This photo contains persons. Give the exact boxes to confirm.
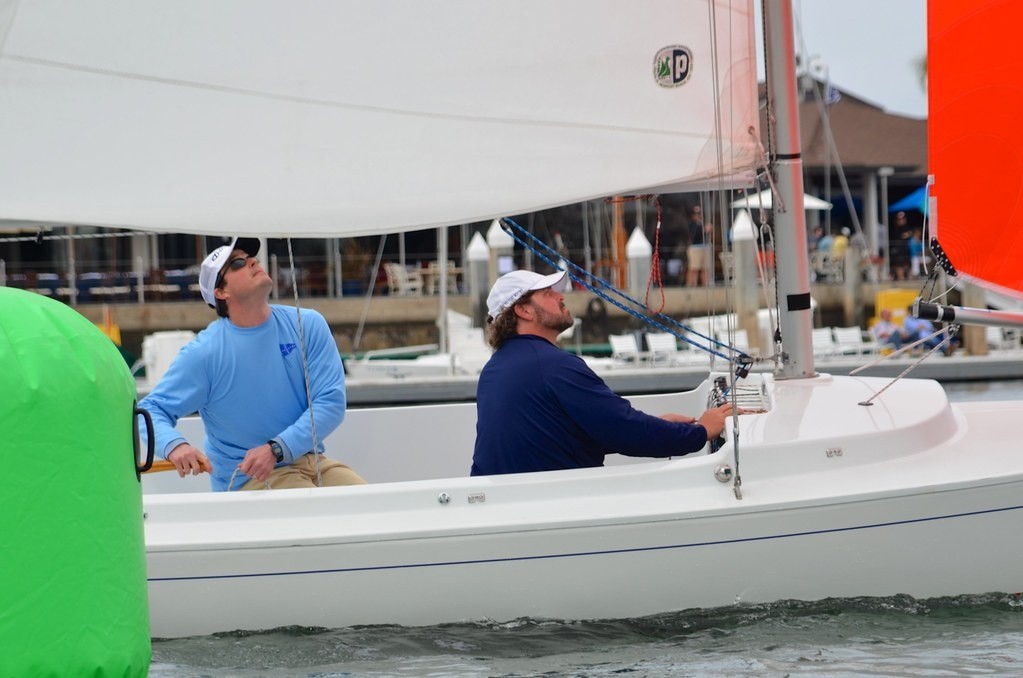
[134,236,366,492]
[469,269,741,477]
[685,202,927,282]
[872,302,959,357]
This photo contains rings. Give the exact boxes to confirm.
[249,470,255,475]
[253,474,258,480]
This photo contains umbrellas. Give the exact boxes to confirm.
[889,185,928,214]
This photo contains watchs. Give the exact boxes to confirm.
[266,440,284,463]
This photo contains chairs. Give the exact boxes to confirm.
[384,258,460,297]
[607,324,880,371]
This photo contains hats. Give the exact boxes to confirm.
[486,270,568,325]
[199,237,260,309]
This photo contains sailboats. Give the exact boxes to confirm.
[0,0,1023,641]
[335,0,1023,411]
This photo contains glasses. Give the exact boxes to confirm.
[215,249,257,289]
